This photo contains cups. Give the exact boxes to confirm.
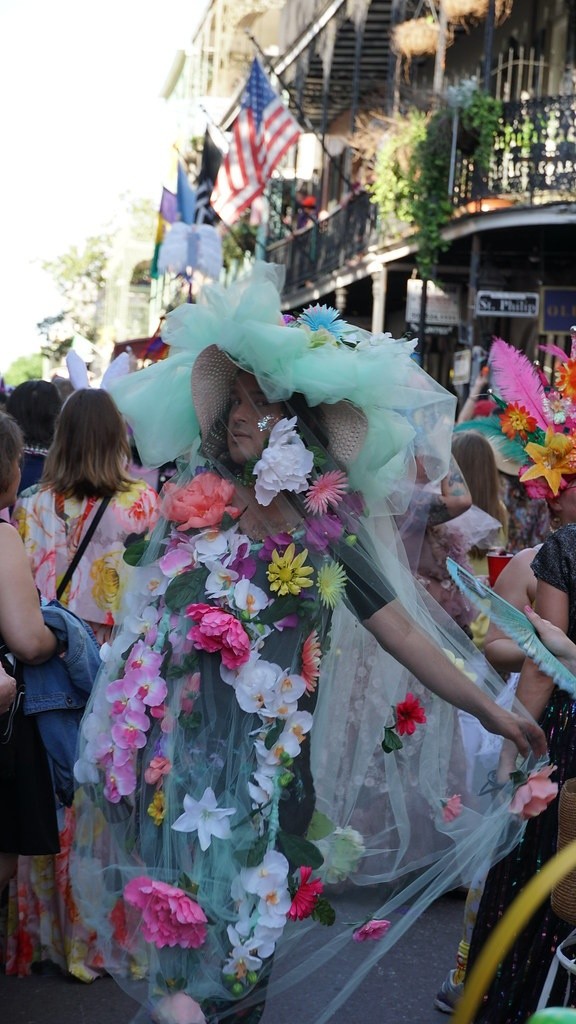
[487,552,514,587]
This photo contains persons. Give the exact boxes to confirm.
[0,255,575,1024]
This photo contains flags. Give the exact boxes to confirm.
[212,61,304,227]
[192,131,224,226]
[174,166,203,268]
[150,189,186,281]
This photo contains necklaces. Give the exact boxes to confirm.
[233,474,254,488]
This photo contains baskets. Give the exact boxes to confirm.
[550,777,576,925]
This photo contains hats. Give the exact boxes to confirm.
[190,306,369,481]
[488,435,528,475]
[454,327,576,500]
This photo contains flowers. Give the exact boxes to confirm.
[471,327,576,500]
[67,415,558,996]
[282,302,394,351]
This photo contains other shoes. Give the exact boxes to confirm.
[433,969,462,1014]
